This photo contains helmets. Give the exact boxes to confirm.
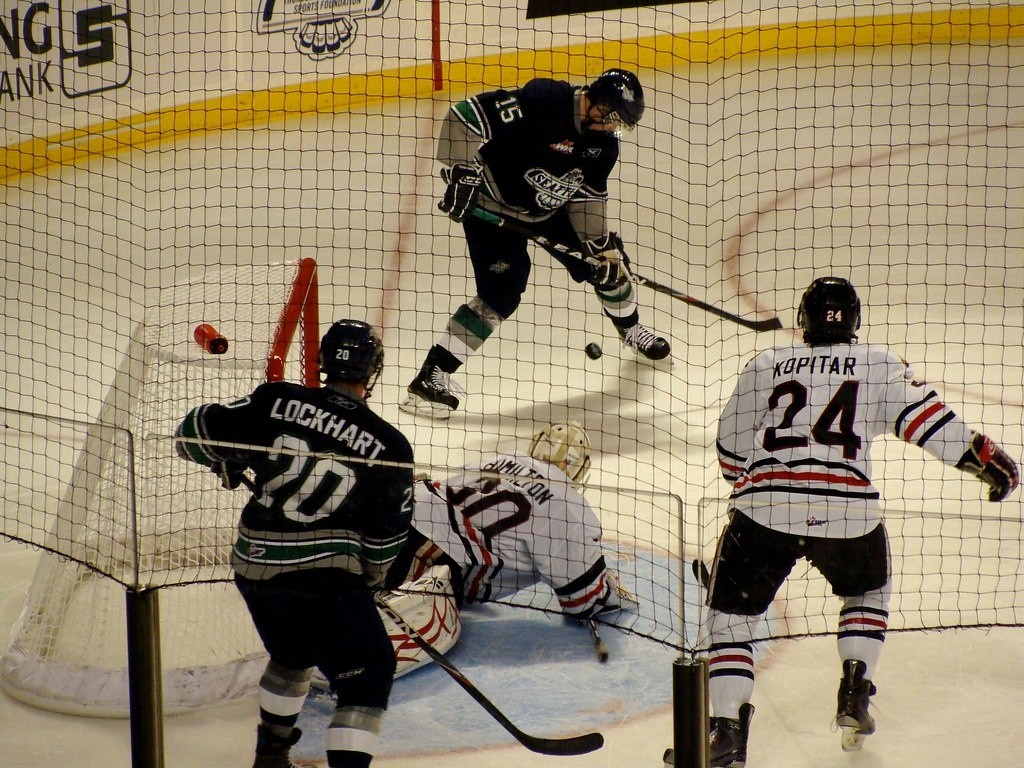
[798,277,861,335]
[316,319,384,391]
[586,68,645,136]
[528,420,591,484]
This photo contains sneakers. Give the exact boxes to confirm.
[252,723,317,768]
[398,345,459,420]
[709,703,755,768]
[837,659,876,751]
[614,323,675,367]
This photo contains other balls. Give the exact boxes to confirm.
[586,343,602,360]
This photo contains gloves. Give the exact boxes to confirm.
[582,231,630,287]
[214,461,248,490]
[437,162,483,223]
[962,433,1019,502]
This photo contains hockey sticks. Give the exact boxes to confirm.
[690,559,710,589]
[208,459,606,757]
[587,619,609,663]
[470,204,801,332]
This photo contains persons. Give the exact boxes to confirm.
[174,318,416,768]
[309,422,638,695]
[663,276,1019,768]
[398,69,672,418]
